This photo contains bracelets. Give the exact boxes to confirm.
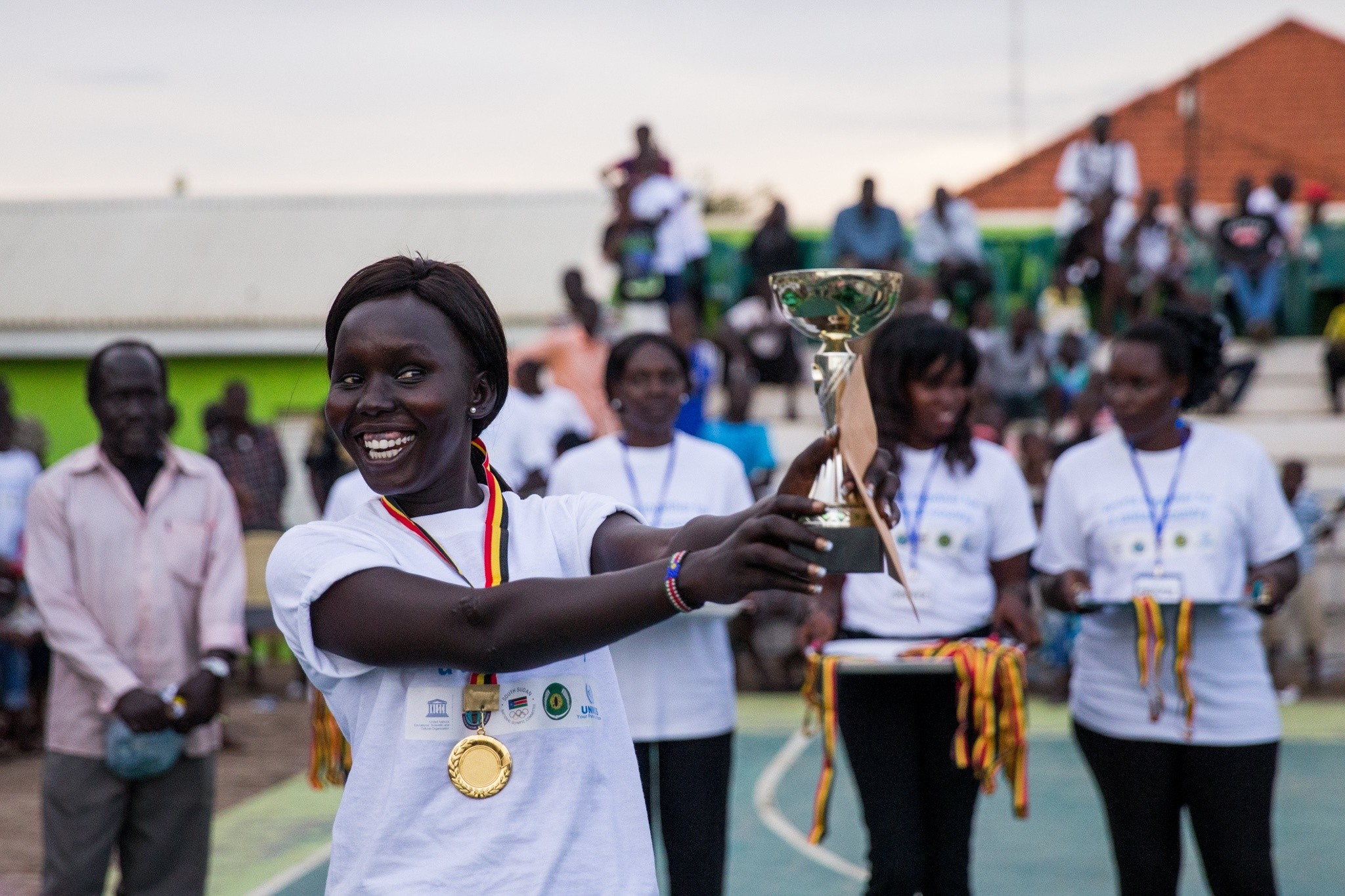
[664,549,705,613]
[196,656,229,682]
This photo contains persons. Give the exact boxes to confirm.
[6,114,1345,896]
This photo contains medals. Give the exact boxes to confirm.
[448,728,512,799]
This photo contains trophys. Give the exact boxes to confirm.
[770,268,904,573]
